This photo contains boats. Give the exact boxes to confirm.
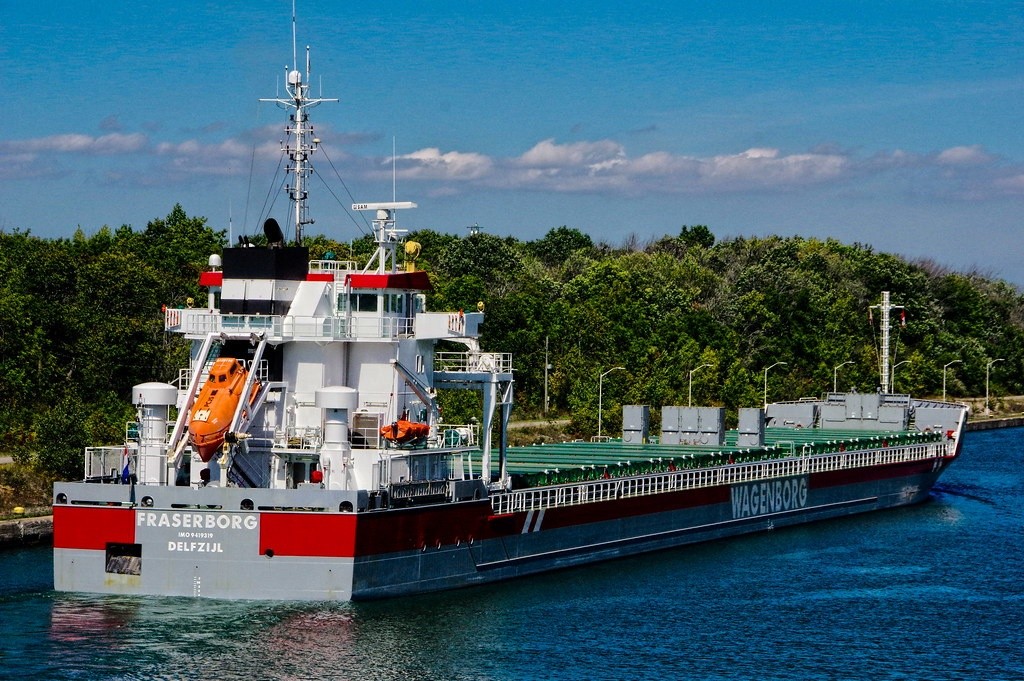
[52,1,969,602]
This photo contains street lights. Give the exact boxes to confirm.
[689,365,712,405]
[834,362,853,394]
[891,361,912,394]
[764,362,786,412]
[943,360,962,403]
[599,367,624,443]
[986,359,1003,414]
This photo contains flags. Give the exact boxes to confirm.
[122,447,129,481]
[901,311,906,328]
